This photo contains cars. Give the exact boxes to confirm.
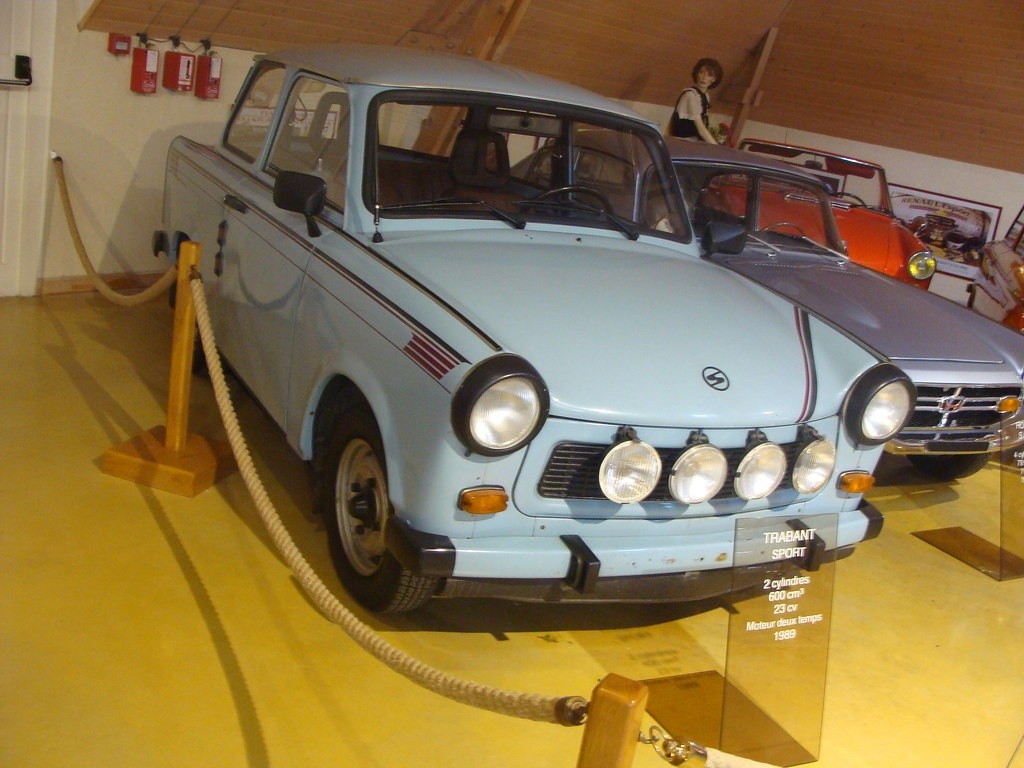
[698,136,939,292]
[152,40,915,619]
[913,214,956,244]
[509,137,1024,483]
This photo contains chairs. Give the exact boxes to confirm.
[446,127,532,213]
[305,89,408,206]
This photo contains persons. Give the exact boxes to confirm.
[671,58,723,145]
